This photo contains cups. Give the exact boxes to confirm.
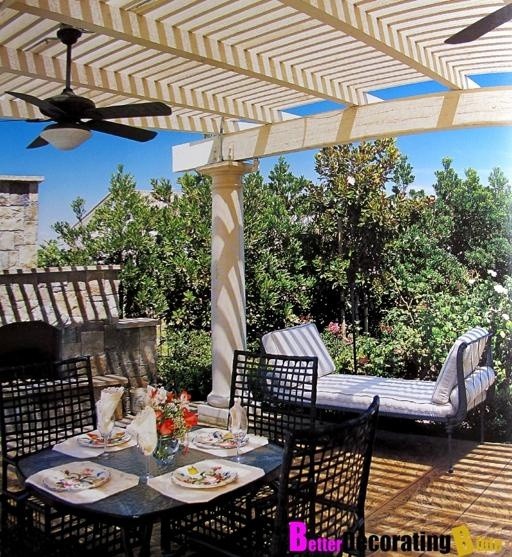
[229,415,247,461]
[137,431,157,483]
[98,415,115,460]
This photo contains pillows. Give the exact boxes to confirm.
[431,327,490,404]
[261,324,337,380]
[451,367,494,407]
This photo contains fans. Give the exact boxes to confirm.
[5,25,172,149]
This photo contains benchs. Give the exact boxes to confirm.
[254,323,497,473]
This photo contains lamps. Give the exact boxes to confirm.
[39,28,95,150]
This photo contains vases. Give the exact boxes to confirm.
[150,435,179,476]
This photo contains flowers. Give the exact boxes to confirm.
[144,388,197,461]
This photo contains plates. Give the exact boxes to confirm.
[192,430,243,450]
[171,462,238,489]
[76,432,131,448]
[42,465,111,492]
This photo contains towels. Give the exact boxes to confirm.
[229,400,249,435]
[96,387,124,430]
[127,407,157,455]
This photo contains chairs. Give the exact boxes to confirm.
[0,355,142,555]
[164,396,381,556]
[168,350,319,521]
